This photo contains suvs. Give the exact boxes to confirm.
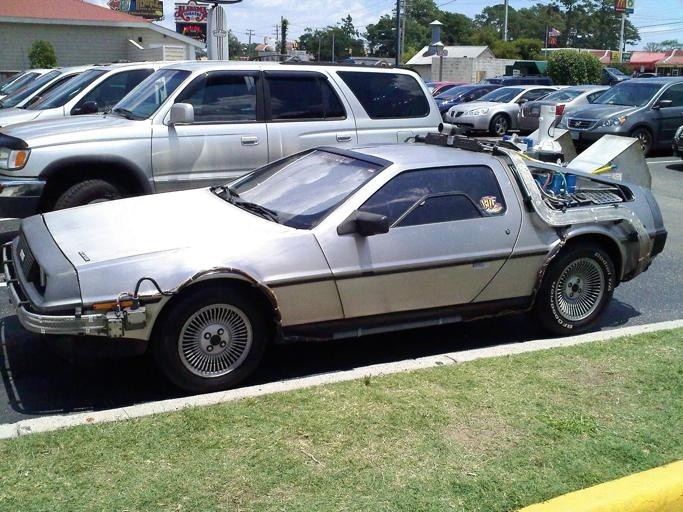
[0,62,112,111]
[560,74,683,156]
[0,60,447,221]
[0,61,283,128]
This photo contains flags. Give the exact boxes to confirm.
[548,27,561,37]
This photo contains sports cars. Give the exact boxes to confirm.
[0,135,665,397]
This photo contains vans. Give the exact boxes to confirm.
[481,75,552,86]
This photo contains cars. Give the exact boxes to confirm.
[635,72,656,78]
[602,67,629,84]
[432,84,505,116]
[424,81,467,97]
[515,84,610,134]
[671,126,682,161]
[442,83,558,136]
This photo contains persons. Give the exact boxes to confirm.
[630,68,639,78]
